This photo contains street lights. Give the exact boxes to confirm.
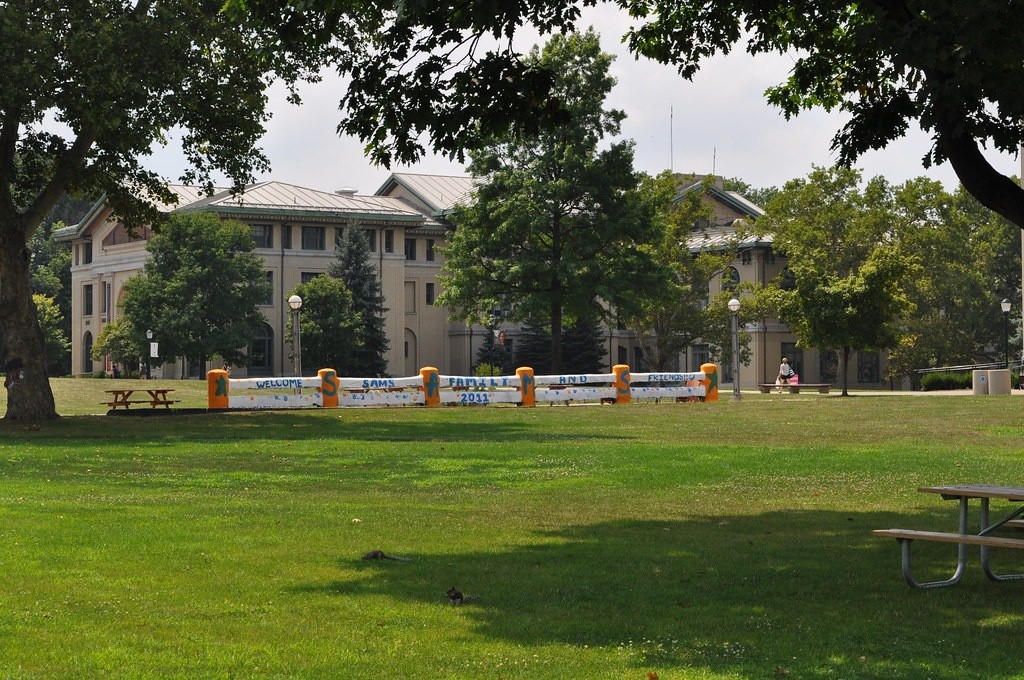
[1001,298,1011,368]
[287,295,304,393]
[728,299,742,400]
[146,329,153,378]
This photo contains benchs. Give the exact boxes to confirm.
[871,528,1024,554]
[101,399,177,407]
[755,383,832,395]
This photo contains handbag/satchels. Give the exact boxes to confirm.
[774,378,784,390]
[789,368,794,377]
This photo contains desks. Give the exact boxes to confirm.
[872,483,1024,594]
[99,388,178,414]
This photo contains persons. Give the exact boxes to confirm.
[112,365,118,379]
[222,362,228,371]
[777,357,791,393]
[227,363,231,377]
[139,362,147,379]
